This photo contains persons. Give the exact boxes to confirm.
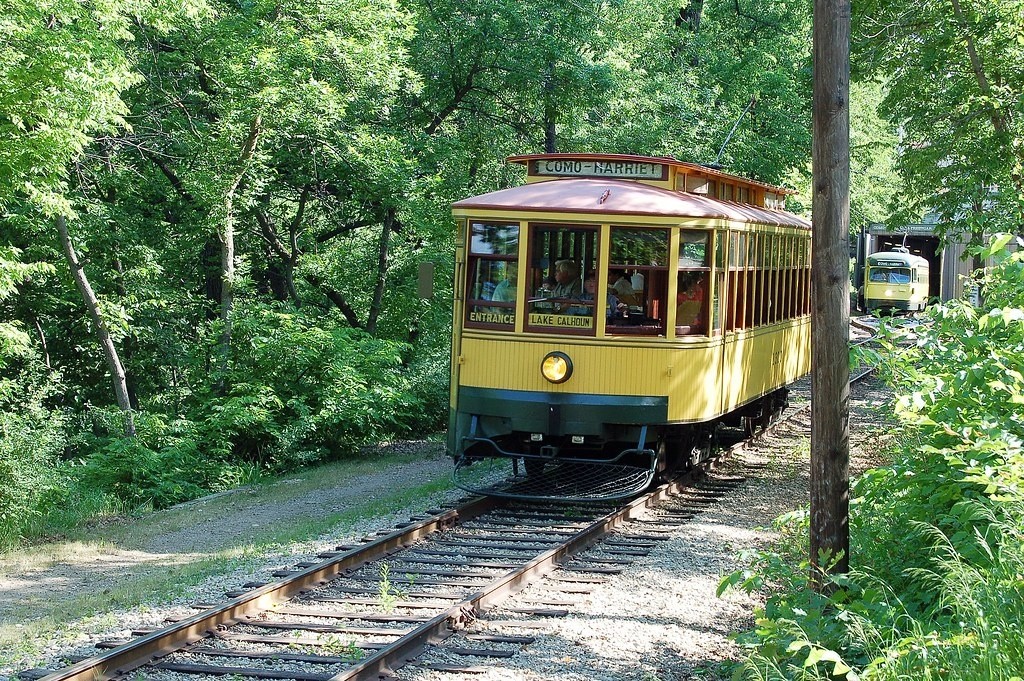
[490,263,518,314]
[542,260,644,316]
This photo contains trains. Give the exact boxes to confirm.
[445,97,813,502]
[863,233,930,314]
